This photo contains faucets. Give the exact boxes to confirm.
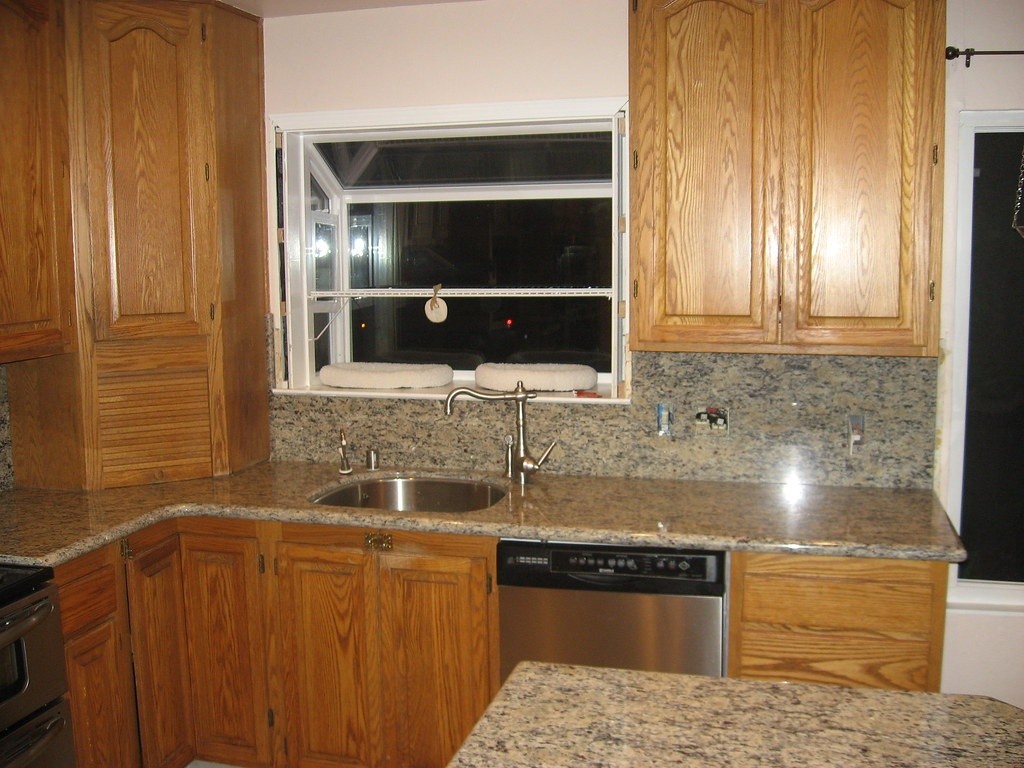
[444,381,556,484]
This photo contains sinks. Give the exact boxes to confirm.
[311,475,508,512]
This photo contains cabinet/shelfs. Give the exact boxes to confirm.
[274,520,493,767]
[57,520,194,768]
[628,1,948,358]
[729,552,947,690]
[184,519,273,767]
[1,1,272,494]
